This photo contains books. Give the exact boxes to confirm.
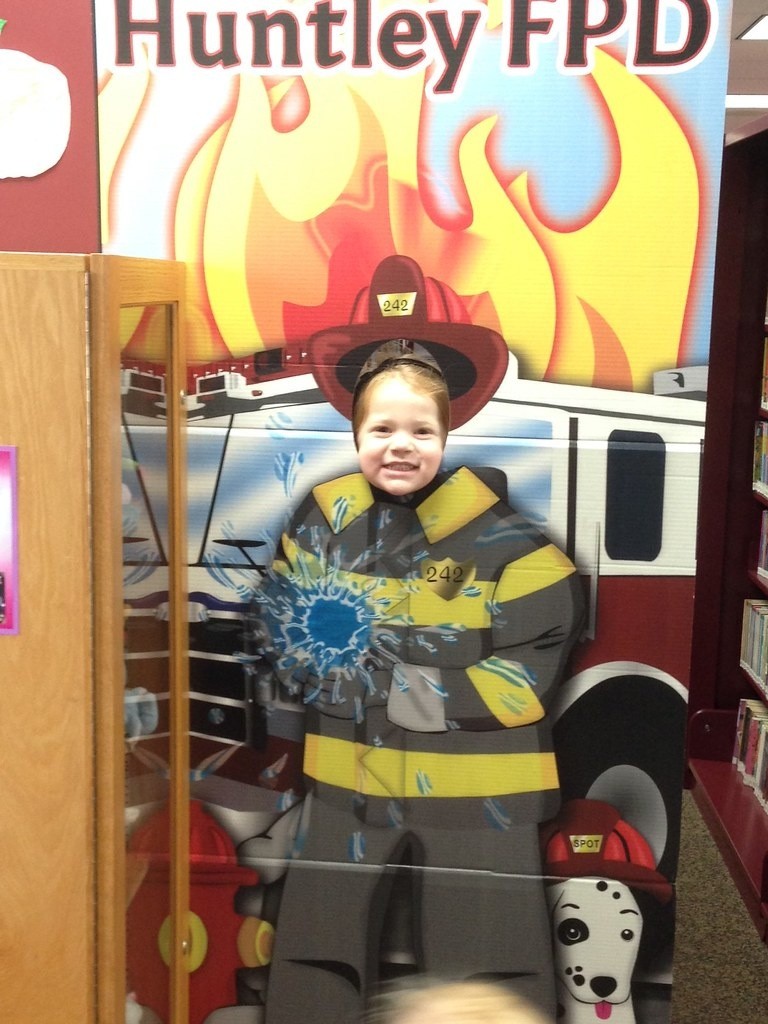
[730,699,768,812]
[756,511,767,578]
[739,600,768,692]
[759,296,768,415]
[753,422,768,497]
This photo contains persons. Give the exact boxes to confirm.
[352,360,451,496]
